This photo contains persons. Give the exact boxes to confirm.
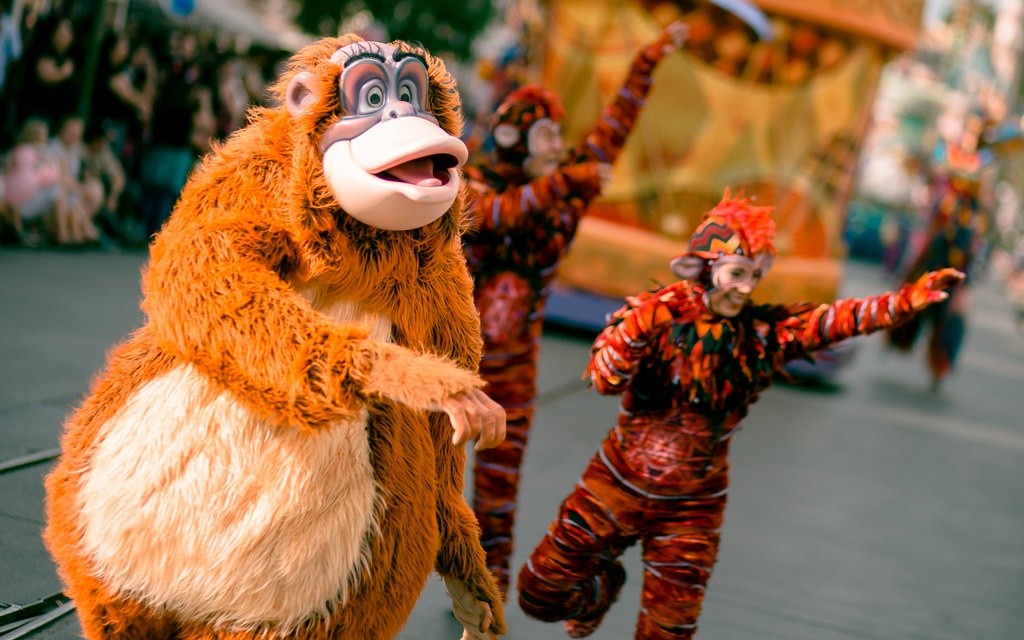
[866,90,1006,398]
[459,22,693,607]
[516,185,967,640]
[0,0,288,251]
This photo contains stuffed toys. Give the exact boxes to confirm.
[40,32,517,640]
